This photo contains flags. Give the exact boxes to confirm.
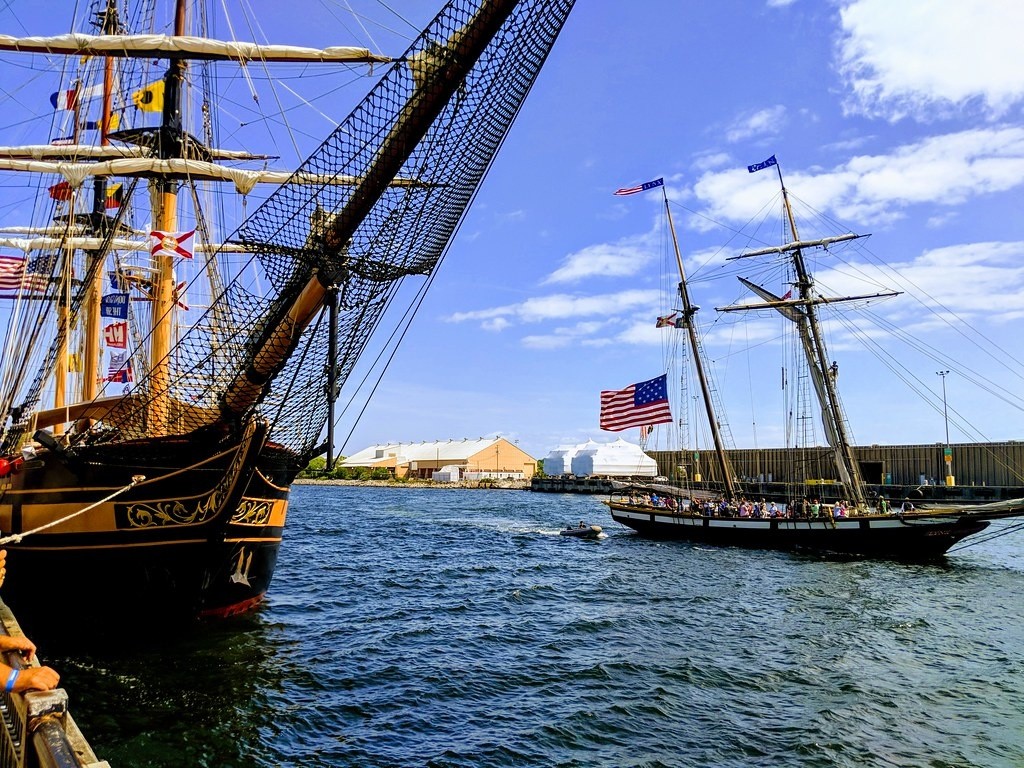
[599,374,673,432]
[747,154,779,174]
[655,311,677,329]
[614,178,664,196]
[781,290,791,301]
[0,77,196,384]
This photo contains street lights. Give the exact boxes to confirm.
[691,395,699,474]
[935,370,952,476]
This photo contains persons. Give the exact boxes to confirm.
[579,521,586,529]
[828,361,839,389]
[629,491,914,519]
[0,548,60,692]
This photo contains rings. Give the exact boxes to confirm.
[0,570,5,577]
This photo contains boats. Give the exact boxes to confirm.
[0,0,579,629]
[599,154,992,561]
[559,525,602,539]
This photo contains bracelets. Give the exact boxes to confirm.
[5,670,19,692]
